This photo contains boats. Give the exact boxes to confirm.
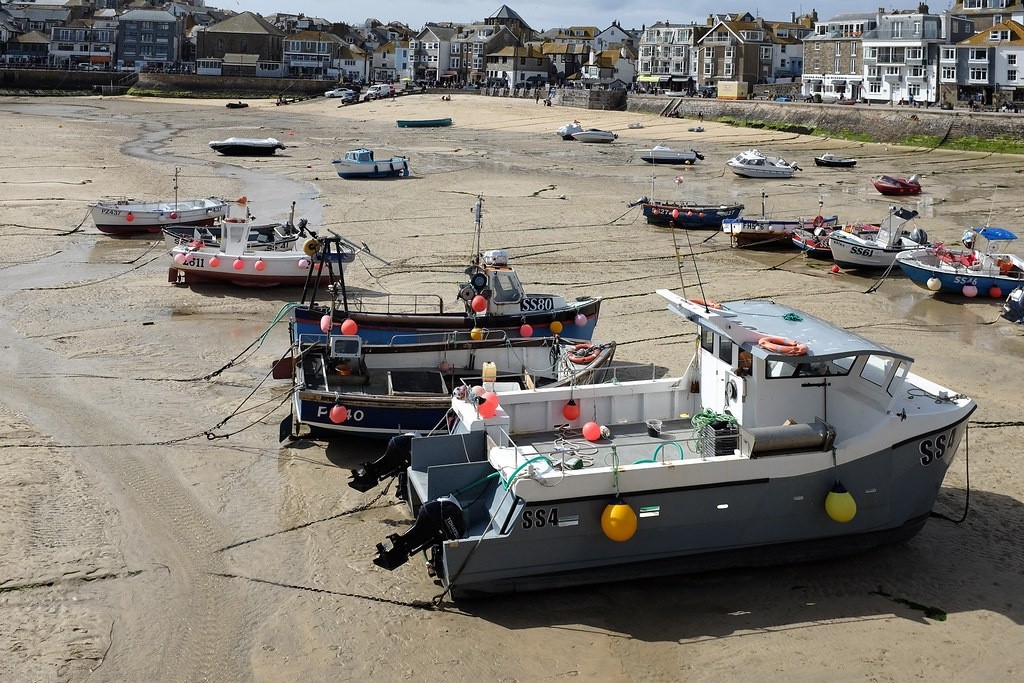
[776,97,792,102]
[688,126,704,132]
[827,203,940,275]
[628,122,645,129]
[870,172,923,196]
[160,198,308,259]
[396,116,453,128]
[605,149,746,231]
[838,99,856,105]
[394,215,979,606]
[571,129,618,145]
[720,186,838,252]
[208,137,287,157]
[894,181,1024,327]
[632,140,707,165]
[813,152,859,169]
[287,190,603,350]
[334,143,417,181]
[665,87,689,98]
[226,101,249,108]
[271,328,618,450]
[790,192,911,261]
[168,217,369,292]
[729,151,804,179]
[86,164,229,236]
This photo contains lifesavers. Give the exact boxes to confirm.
[812,215,824,228]
[567,343,600,363]
[759,336,809,355]
[688,298,723,310]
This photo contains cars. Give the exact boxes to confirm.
[324,87,353,98]
[78,62,118,72]
[941,102,954,110]
[340,90,360,104]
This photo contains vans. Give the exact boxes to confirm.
[367,84,391,101]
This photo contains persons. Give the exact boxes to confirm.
[442,95,451,101]
[535,94,551,106]
[1003,100,1014,109]
[383,78,393,85]
[698,113,703,122]
[354,76,365,89]
[703,90,711,98]
[631,84,635,94]
[908,94,913,107]
[968,99,979,111]
[443,81,473,89]
[405,81,408,90]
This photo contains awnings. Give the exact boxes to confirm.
[803,79,861,85]
